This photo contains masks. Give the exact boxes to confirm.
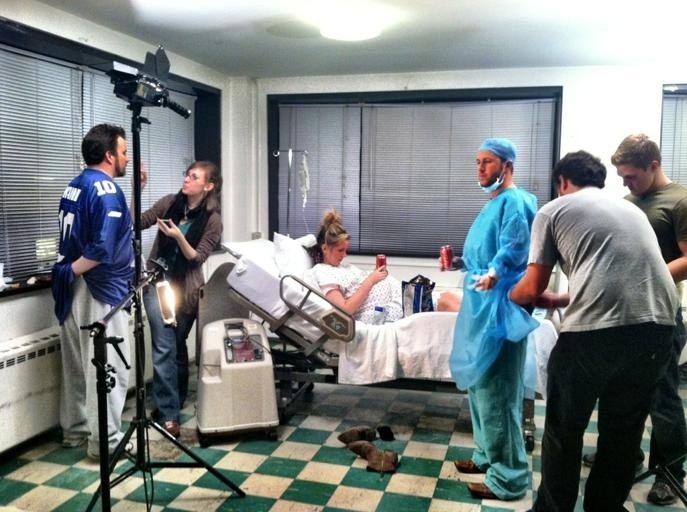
[478,169,504,193]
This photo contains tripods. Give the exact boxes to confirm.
[84,298,246,512]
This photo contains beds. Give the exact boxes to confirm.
[200,237,561,453]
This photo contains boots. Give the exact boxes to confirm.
[348,442,399,473]
[338,426,394,444]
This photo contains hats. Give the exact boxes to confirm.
[481,138,517,163]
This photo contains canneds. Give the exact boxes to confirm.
[440,245,453,269]
[376,253,386,270]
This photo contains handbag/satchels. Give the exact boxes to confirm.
[401,275,435,317]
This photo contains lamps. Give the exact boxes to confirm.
[78,258,177,511]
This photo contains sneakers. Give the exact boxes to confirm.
[63,437,88,447]
[87,433,138,458]
[163,420,180,438]
[468,483,495,497]
[583,451,642,473]
[454,460,481,472]
[647,477,683,505]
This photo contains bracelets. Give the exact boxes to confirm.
[365,277,373,286]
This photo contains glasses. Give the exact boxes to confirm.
[183,171,199,181]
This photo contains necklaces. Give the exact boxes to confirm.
[184,203,195,221]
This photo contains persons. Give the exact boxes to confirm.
[581,132,687,507]
[311,205,462,326]
[48,120,137,463]
[504,146,680,512]
[438,133,538,501]
[128,159,225,441]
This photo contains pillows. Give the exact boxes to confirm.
[272,232,319,279]
